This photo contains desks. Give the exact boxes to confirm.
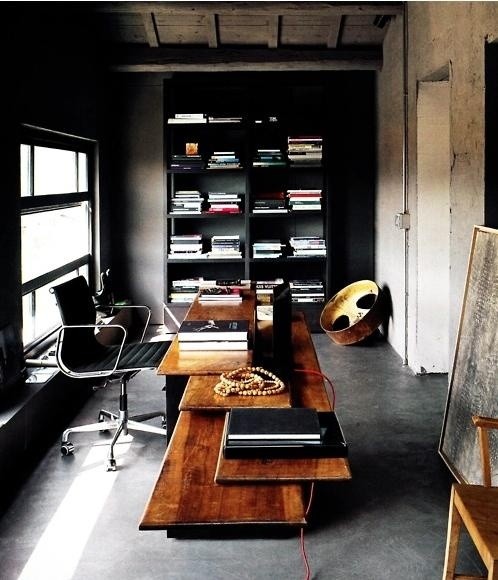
[138,288,353,539]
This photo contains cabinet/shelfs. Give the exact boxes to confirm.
[161,76,248,308]
[248,77,332,305]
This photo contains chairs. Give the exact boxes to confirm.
[443,416,497,580]
[50,274,172,471]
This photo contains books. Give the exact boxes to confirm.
[167,112,327,307]
[222,407,349,459]
[177,340,248,351]
[176,318,250,342]
